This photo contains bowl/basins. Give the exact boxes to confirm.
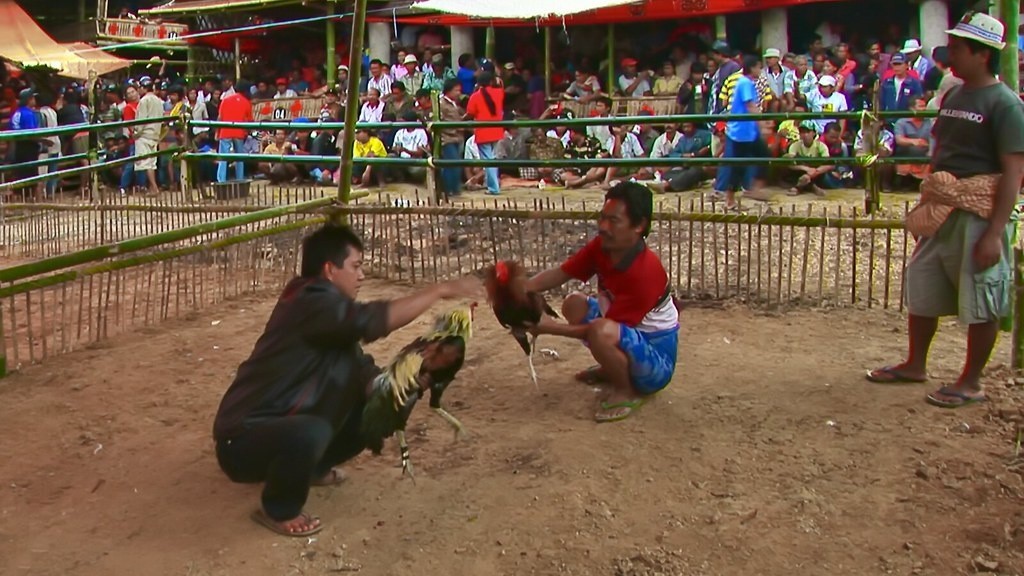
[213,179,254,199]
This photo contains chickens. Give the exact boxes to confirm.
[478,259,562,388]
[358,299,478,486]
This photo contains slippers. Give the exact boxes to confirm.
[595,397,646,422]
[866,365,928,384]
[251,509,324,537]
[575,365,608,383]
[309,467,347,486]
[927,386,984,408]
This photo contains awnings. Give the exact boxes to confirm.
[0,0,131,78]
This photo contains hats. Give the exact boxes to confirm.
[762,48,780,58]
[20,76,169,98]
[815,75,835,87]
[505,62,514,70]
[620,58,638,67]
[798,119,815,130]
[324,88,338,96]
[712,41,730,56]
[899,39,922,53]
[275,78,288,84]
[338,65,349,72]
[944,10,1006,50]
[403,54,418,66]
[889,53,909,64]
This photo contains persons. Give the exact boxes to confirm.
[506,181,679,424]
[865,10,1024,406]
[0,38,963,198]
[211,223,490,536]
